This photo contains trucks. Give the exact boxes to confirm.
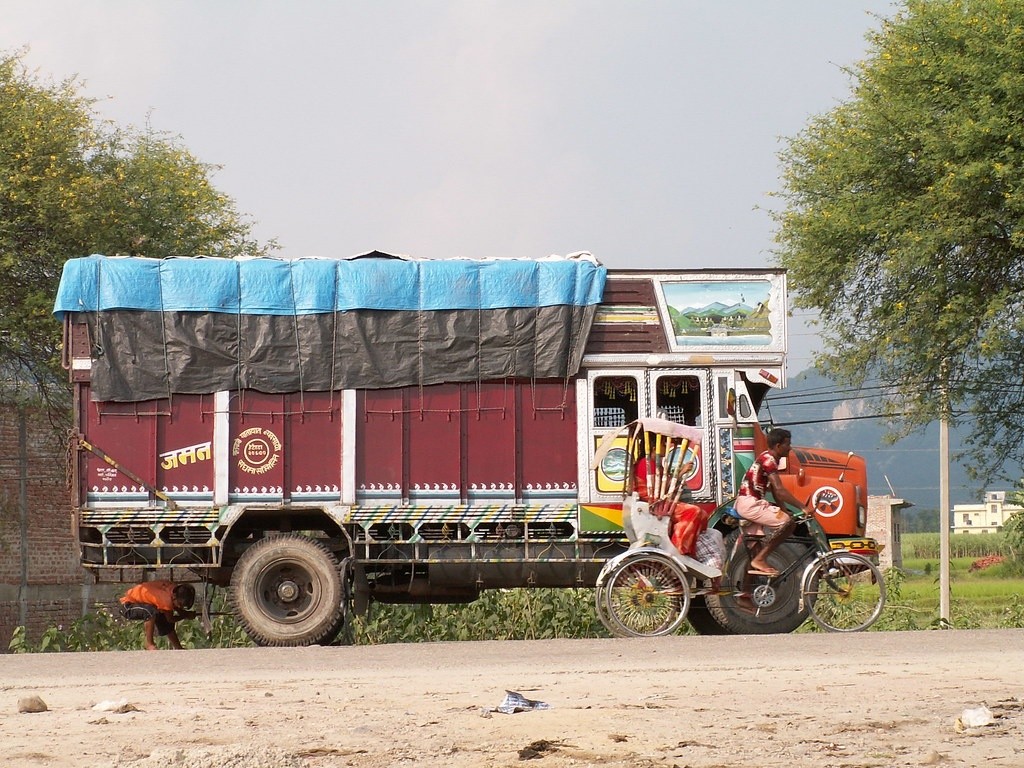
[61,264,867,647]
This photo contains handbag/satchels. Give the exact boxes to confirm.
[695,528,727,570]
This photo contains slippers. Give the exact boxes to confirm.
[748,567,779,575]
[734,601,761,616]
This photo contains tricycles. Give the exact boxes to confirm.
[595,417,889,637]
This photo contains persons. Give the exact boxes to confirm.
[733,428,811,576]
[633,432,708,557]
[118,580,197,650]
